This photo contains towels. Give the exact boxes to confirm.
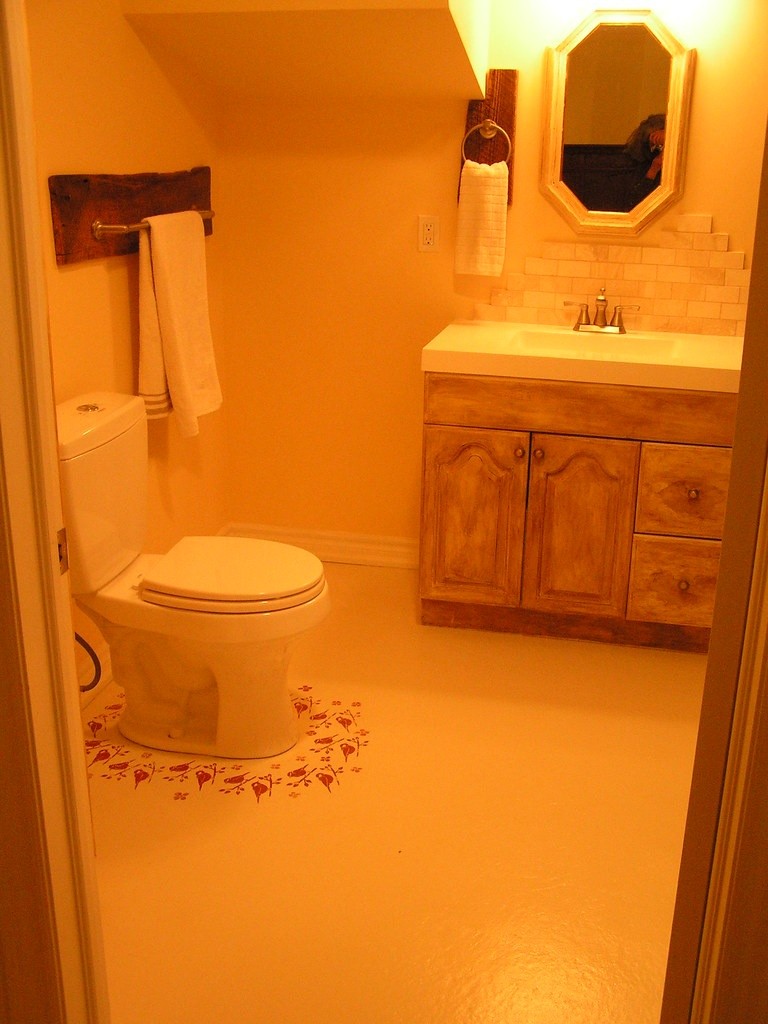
[136,207,224,421]
[454,158,510,277]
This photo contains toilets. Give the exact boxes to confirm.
[54,382,333,761]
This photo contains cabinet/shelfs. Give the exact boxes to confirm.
[416,319,744,650]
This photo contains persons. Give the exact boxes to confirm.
[623,114,667,208]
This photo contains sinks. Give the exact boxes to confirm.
[419,321,743,392]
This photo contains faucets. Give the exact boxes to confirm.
[563,288,640,334]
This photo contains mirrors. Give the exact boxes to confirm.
[540,9,699,238]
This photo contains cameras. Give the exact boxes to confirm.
[652,144,664,156]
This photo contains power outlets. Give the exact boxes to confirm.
[417,215,440,253]
